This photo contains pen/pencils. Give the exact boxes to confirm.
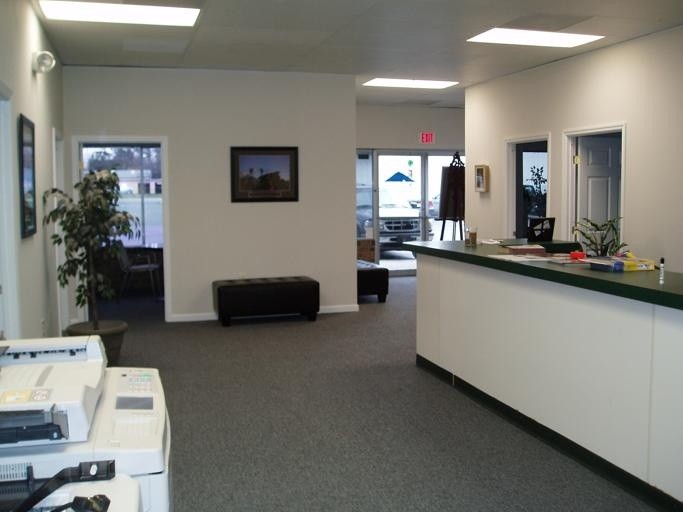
[659,258,664,280]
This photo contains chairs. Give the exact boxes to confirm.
[111,239,163,303]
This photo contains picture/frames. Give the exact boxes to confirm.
[474,164,489,193]
[18,113,37,240]
[230,147,299,203]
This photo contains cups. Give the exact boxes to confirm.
[464,225,477,247]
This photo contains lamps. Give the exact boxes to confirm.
[32,50,56,73]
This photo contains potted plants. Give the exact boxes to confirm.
[40,167,142,368]
[527,164,546,217]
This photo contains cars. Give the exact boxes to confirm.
[355,185,434,243]
[427,194,441,217]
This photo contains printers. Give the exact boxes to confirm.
[0,335,172,511]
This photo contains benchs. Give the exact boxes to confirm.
[213,275,320,327]
[357,258,389,305]
